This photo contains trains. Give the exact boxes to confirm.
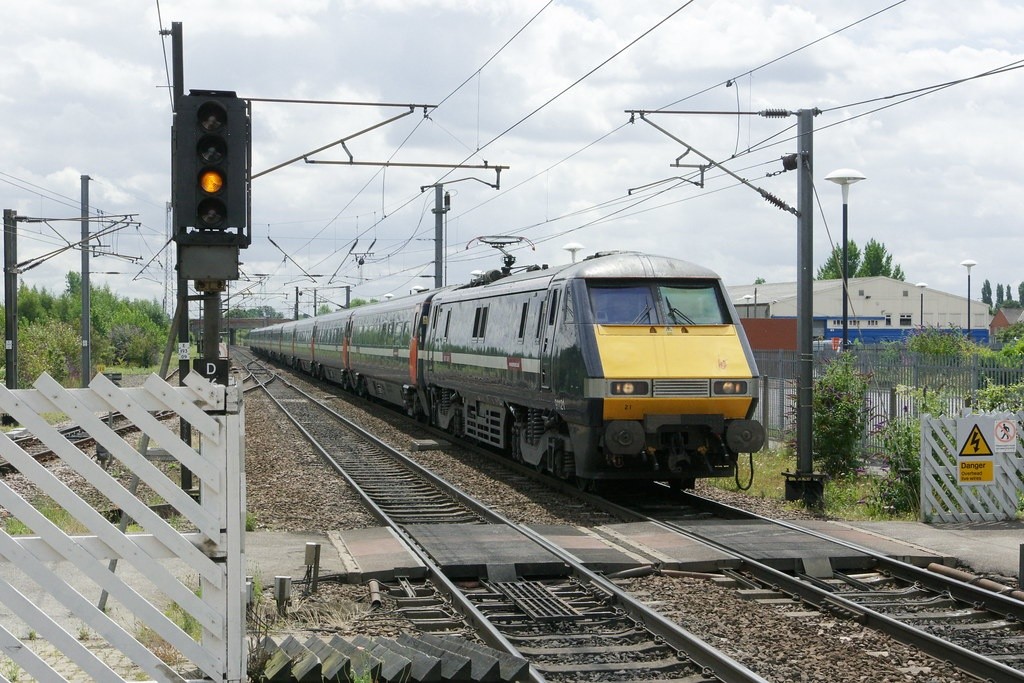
[243,251,766,492]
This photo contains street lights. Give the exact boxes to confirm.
[825,168,867,352]
[564,243,586,263]
[915,282,928,331]
[744,295,754,317]
[961,260,977,340]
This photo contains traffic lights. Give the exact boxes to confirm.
[173,90,251,248]
